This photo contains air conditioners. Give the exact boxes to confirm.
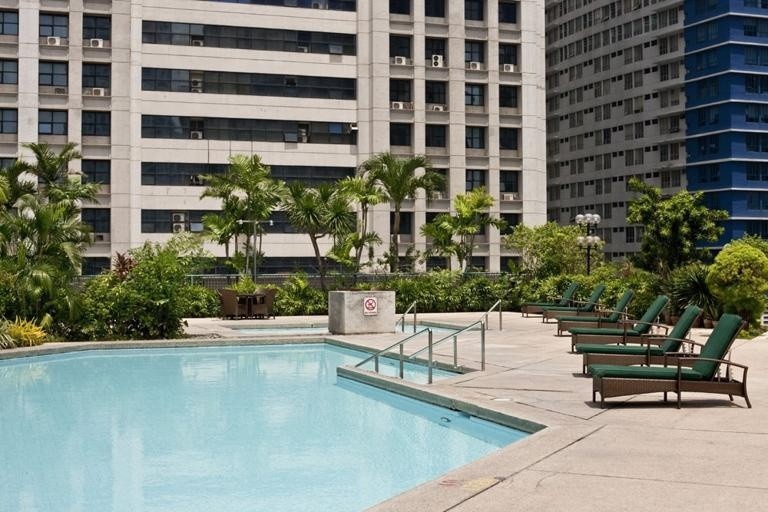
[290,46,308,53]
[173,223,185,232]
[193,39,203,46]
[504,194,514,201]
[298,129,306,136]
[431,191,442,199]
[390,234,400,243]
[191,131,203,139]
[297,136,307,143]
[312,0,326,9]
[47,37,60,45]
[173,213,185,221]
[191,79,203,92]
[466,192,474,196]
[93,88,104,96]
[391,55,514,112]
[90,38,103,48]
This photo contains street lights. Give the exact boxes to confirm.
[573,211,603,276]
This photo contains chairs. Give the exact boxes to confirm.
[219,286,276,320]
[522,283,751,410]
